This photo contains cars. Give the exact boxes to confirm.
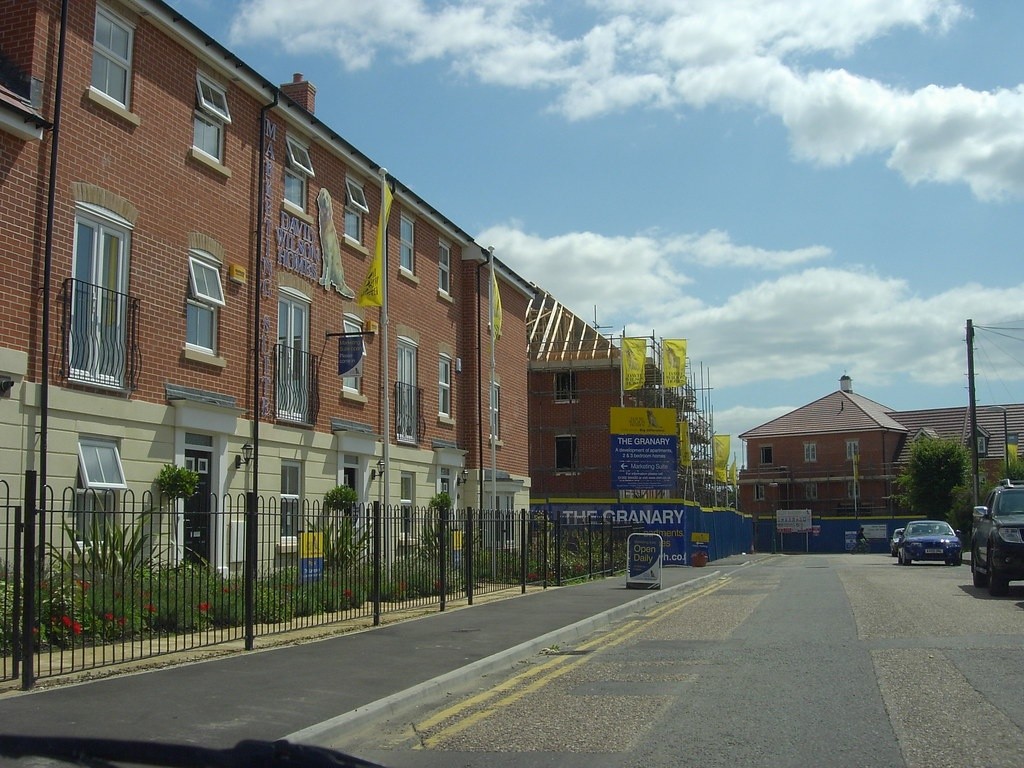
[889,527,904,557]
[897,520,964,567]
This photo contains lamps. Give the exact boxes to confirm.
[372,459,385,480]
[236,441,253,468]
[457,469,469,486]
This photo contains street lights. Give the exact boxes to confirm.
[985,405,1011,479]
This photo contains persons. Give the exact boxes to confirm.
[856,528,868,553]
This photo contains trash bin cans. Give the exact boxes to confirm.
[691,545,708,567]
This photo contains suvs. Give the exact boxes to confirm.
[970,479,1024,597]
